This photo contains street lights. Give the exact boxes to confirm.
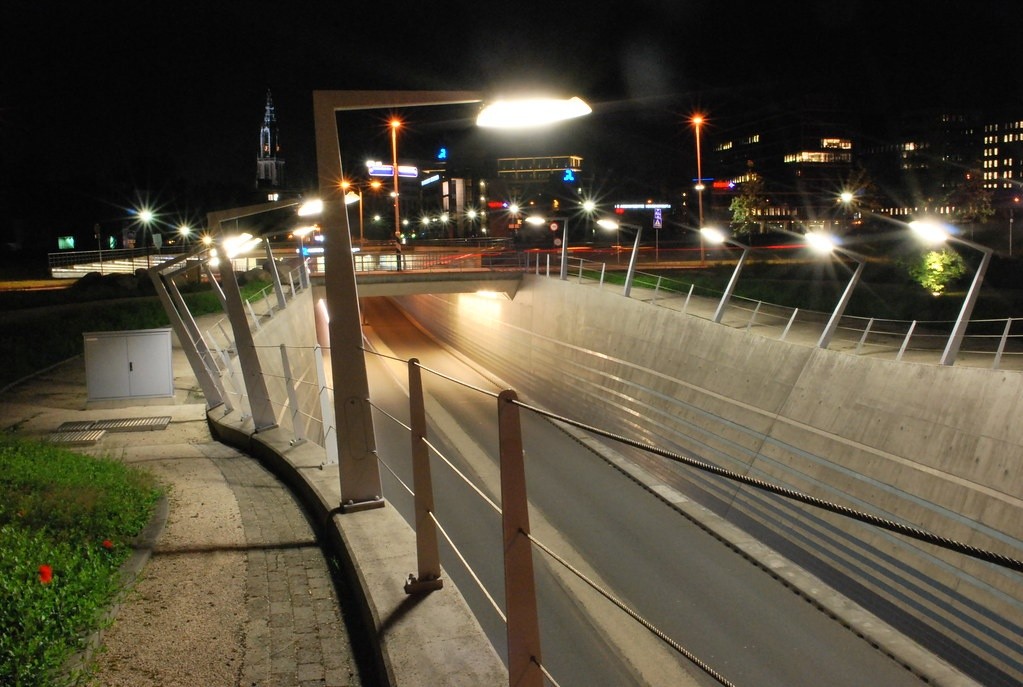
[695,116,705,259]
[313,89,591,513]
[390,119,401,270]
[141,211,151,268]
